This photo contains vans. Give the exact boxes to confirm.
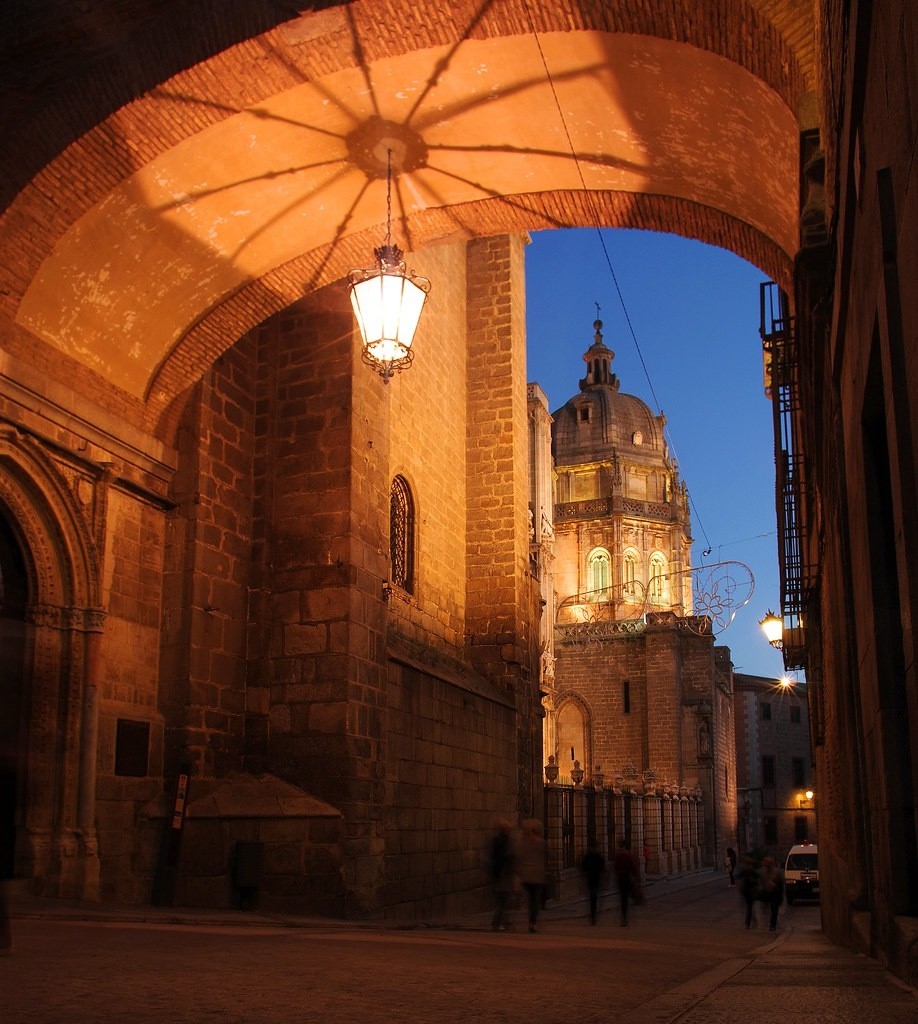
[784,839,820,904]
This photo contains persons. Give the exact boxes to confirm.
[643,843,649,874]
[515,817,548,934]
[610,839,645,926]
[737,854,759,930]
[0,762,16,954]
[488,818,517,932]
[759,857,785,932]
[580,837,608,926]
[726,847,737,887]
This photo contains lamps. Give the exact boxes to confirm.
[346,147,431,383]
[758,608,783,650]
